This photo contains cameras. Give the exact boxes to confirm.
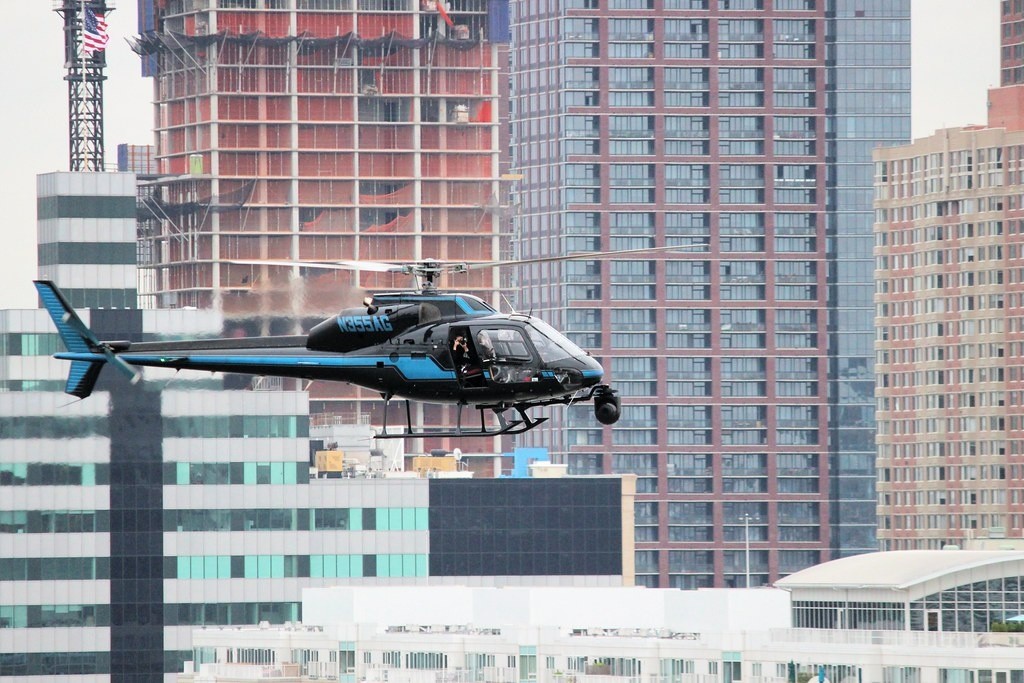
[460,338,466,345]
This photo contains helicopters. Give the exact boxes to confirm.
[32,239,713,437]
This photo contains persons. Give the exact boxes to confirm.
[449,327,481,376]
